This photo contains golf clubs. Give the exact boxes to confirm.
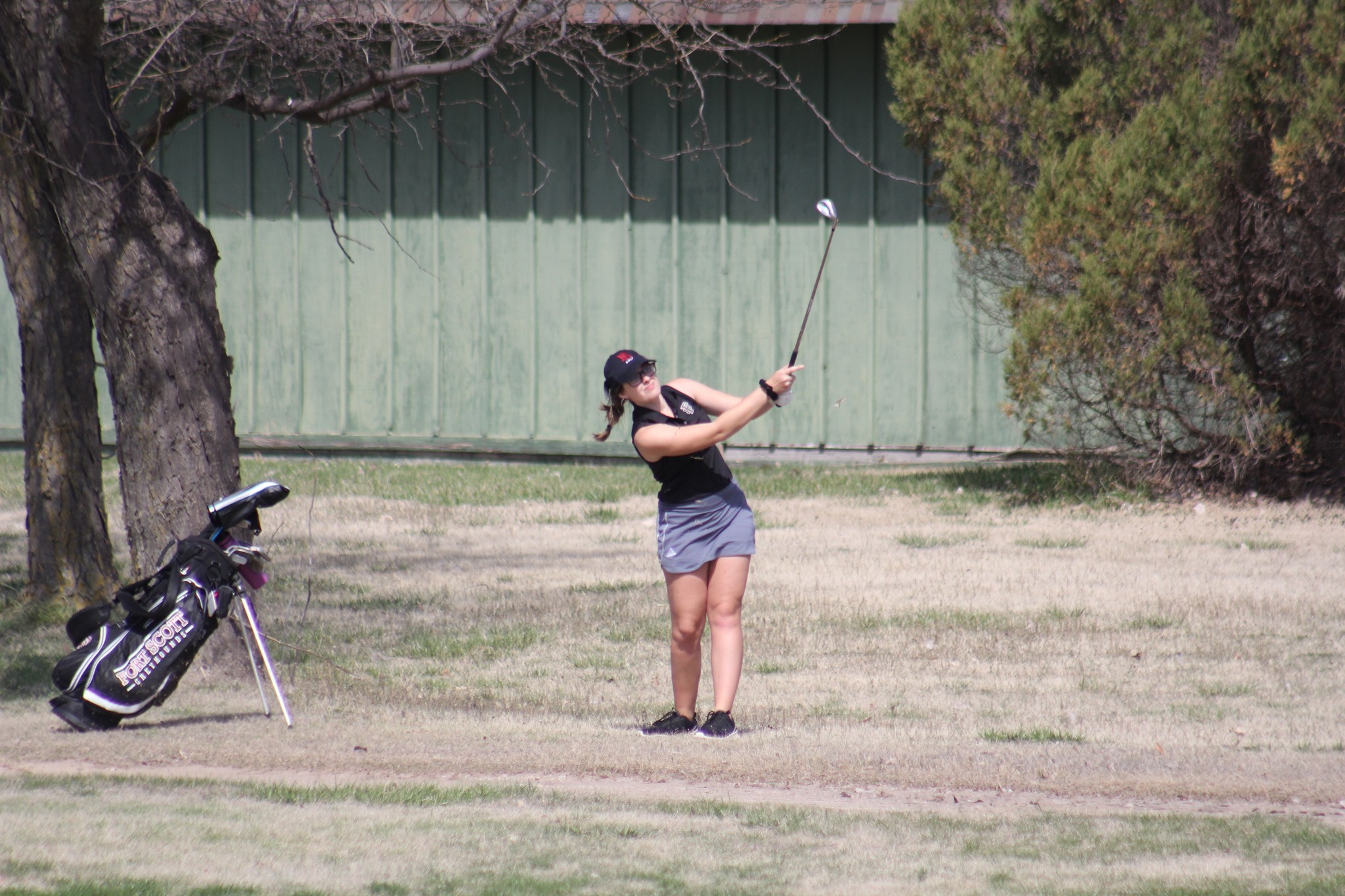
[774,198,839,409]
[210,518,271,568]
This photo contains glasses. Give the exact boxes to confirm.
[620,361,656,388]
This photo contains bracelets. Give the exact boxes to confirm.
[758,380,777,402]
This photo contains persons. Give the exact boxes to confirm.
[593,350,806,740]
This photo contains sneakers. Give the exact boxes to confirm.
[637,707,698,738]
[694,709,737,740]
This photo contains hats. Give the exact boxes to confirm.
[603,349,656,385]
